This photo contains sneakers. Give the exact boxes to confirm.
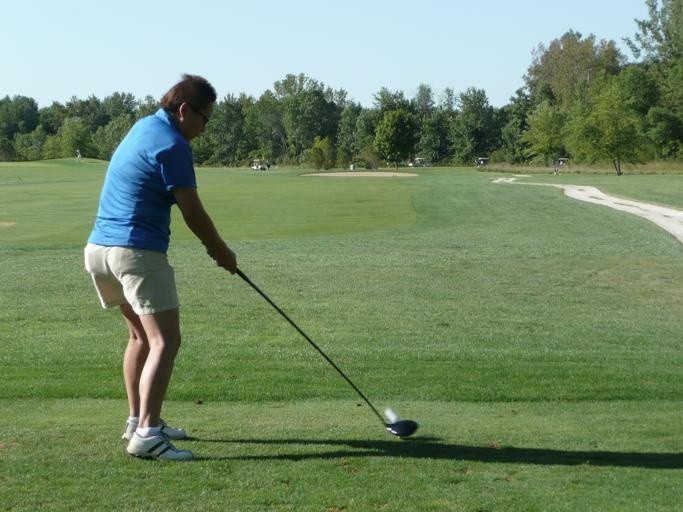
[124,430,195,464]
[119,414,189,441]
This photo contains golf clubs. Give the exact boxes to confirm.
[237,268,404,436]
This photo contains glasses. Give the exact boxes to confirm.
[188,103,209,125]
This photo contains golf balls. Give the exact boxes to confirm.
[388,415,401,424]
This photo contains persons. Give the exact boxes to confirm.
[82,72,238,463]
